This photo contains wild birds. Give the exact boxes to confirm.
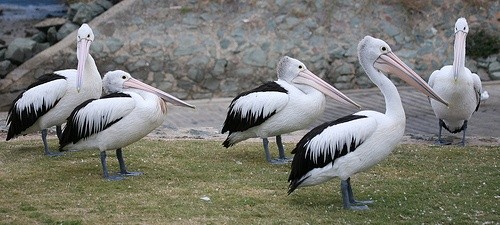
[218,54,364,167]
[56,67,196,181]
[426,15,485,148]
[283,33,450,215]
[4,23,104,160]
